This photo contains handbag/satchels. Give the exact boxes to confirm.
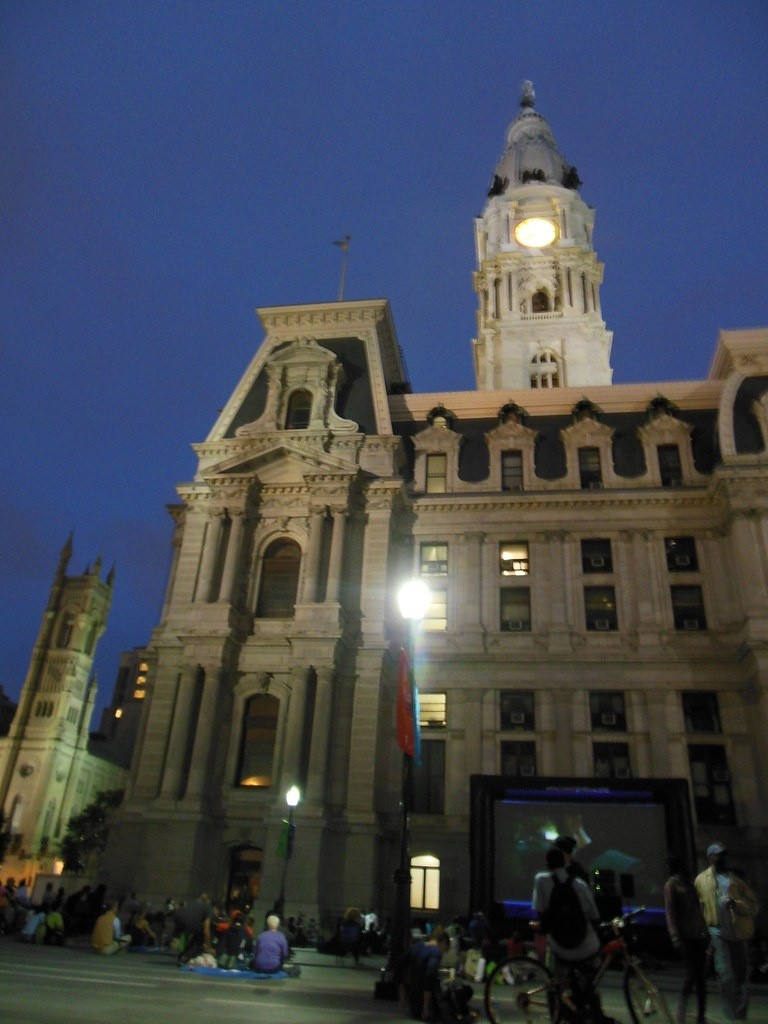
[375,964,401,1002]
[42,926,64,946]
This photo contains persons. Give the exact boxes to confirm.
[532,834,759,1024]
[0,875,537,1022]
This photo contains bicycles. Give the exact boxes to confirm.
[482,905,677,1024]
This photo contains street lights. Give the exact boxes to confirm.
[395,580,434,814]
[276,784,300,916]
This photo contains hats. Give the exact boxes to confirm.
[707,841,726,856]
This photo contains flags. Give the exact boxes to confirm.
[395,647,422,770]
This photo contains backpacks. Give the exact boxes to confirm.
[544,871,587,951]
